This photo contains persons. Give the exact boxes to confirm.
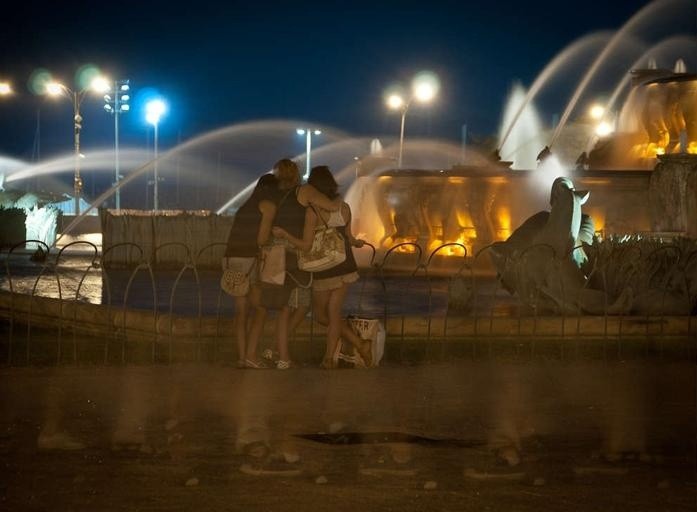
[219,159,372,371]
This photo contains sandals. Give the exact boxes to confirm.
[237,350,292,370]
[357,339,372,367]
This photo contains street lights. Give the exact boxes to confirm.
[97,77,133,210]
[42,71,104,214]
[296,127,322,178]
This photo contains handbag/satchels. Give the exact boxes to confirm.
[343,317,386,370]
[297,204,346,272]
[259,244,290,310]
[220,271,250,297]
